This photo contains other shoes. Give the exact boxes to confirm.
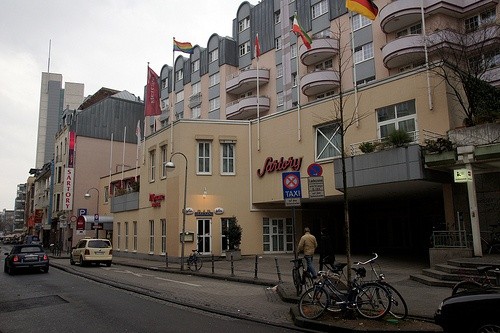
[317,274,321,276]
[312,276,317,279]
[305,274,308,277]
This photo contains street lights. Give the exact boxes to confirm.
[165,151,188,272]
[84,187,99,239]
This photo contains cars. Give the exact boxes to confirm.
[433,287,500,333]
[4,244,49,276]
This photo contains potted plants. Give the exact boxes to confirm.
[224,215,242,260]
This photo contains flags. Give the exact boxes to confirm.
[135,122,142,142]
[173,37,194,55]
[345,0,379,21]
[144,67,161,117]
[292,12,313,50]
[255,32,261,61]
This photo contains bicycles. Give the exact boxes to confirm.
[289,251,409,320]
[187,249,203,272]
[451,265,500,297]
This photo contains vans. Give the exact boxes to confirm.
[69,236,113,267]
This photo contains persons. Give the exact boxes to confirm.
[297,227,317,279]
[318,226,335,272]
